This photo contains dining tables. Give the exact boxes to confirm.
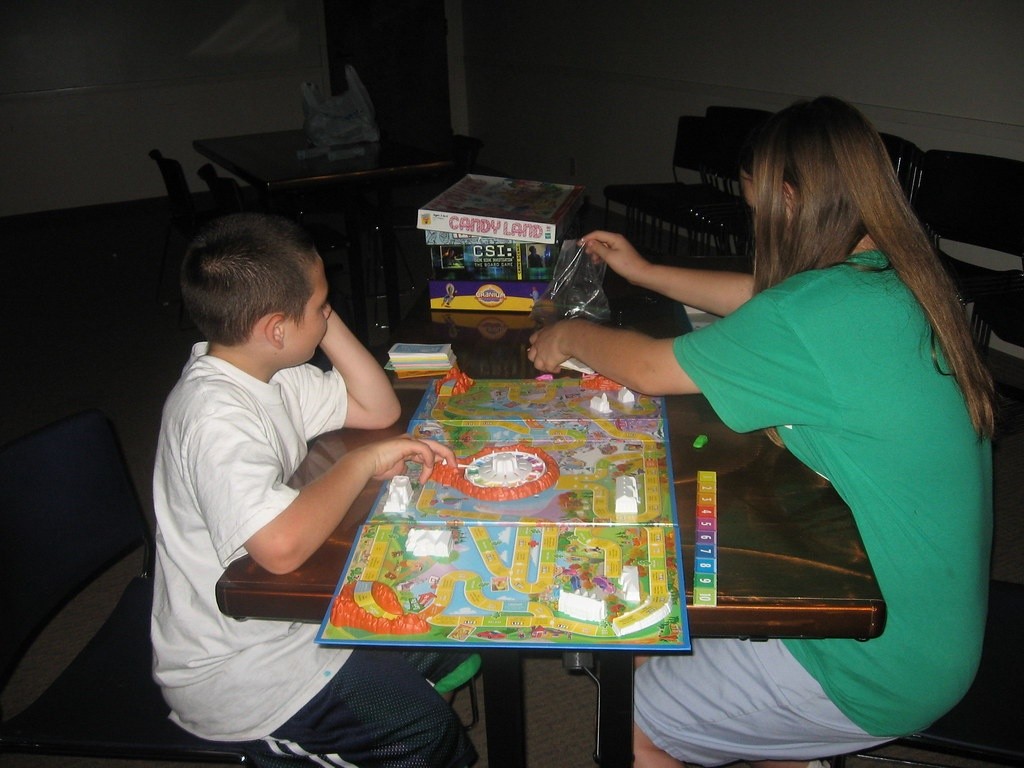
[192,127,457,349]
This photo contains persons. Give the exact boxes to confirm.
[148,218,488,768]
[527,95,995,768]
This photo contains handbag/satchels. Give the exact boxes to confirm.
[300,66,380,142]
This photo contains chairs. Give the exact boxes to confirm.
[196,162,349,254]
[829,576,1023,768]
[600,103,1023,369]
[148,148,226,305]
[3,407,260,768]
[362,134,484,330]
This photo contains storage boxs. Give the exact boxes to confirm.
[415,172,587,315]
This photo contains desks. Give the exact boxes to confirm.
[213,274,888,768]
